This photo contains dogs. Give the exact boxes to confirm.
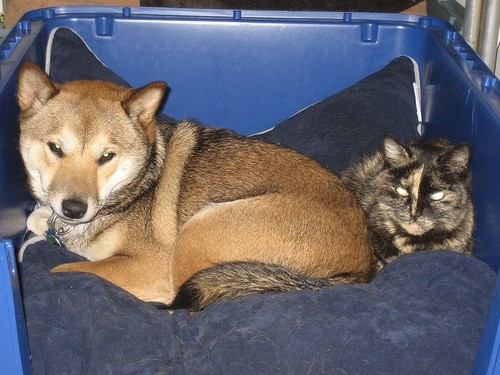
[17,60,376,312]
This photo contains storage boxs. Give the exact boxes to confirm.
[0,6,498,375]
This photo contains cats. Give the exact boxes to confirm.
[340,133,473,272]
